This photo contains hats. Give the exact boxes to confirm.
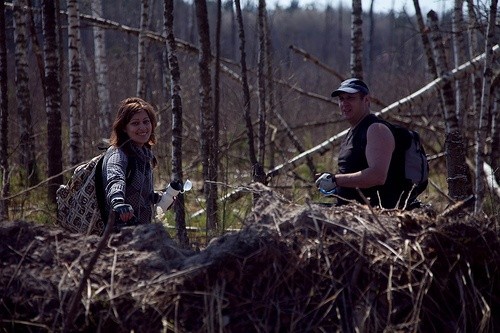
[330,78,370,95]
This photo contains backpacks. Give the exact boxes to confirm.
[55,151,111,238]
[381,119,429,210]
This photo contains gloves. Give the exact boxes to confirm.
[315,171,336,197]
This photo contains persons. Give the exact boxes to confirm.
[314,76,409,210]
[100,97,164,233]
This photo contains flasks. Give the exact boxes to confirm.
[154,177,193,215]
